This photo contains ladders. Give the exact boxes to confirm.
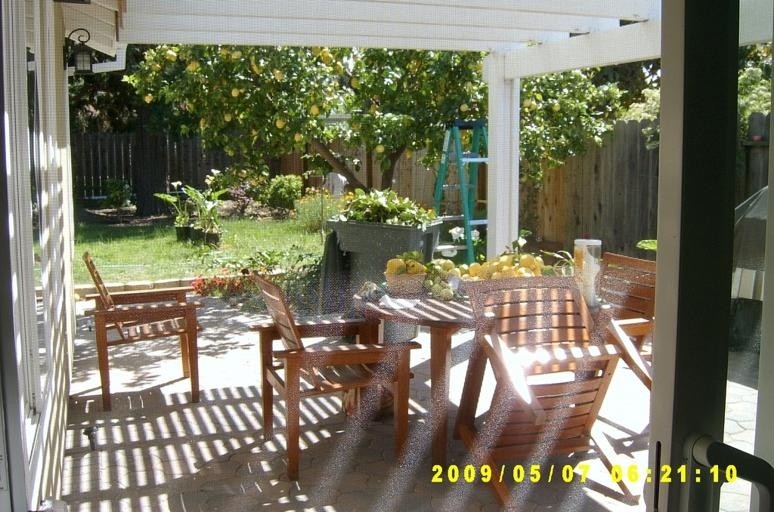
[432,119,489,267]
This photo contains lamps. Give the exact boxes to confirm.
[62,26,93,75]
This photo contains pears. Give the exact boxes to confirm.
[143,44,560,189]
[387,253,544,300]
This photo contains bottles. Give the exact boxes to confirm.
[574,239,604,308]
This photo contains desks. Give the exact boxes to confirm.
[352,288,480,470]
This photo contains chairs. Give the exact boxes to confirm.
[245,269,421,480]
[591,250,658,360]
[77,250,205,411]
[455,275,654,511]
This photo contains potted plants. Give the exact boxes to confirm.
[324,184,445,258]
[153,179,222,246]
